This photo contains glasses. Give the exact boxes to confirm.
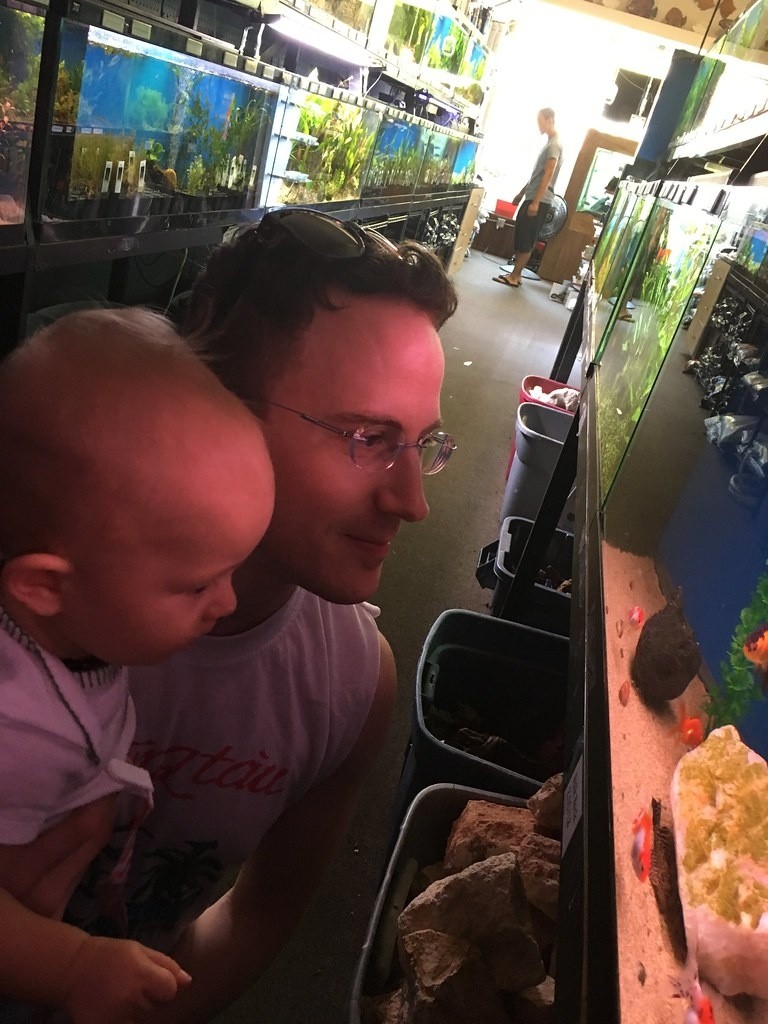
[242,397,455,474]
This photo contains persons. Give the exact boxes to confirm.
[1,208,464,1024]
[491,108,565,288]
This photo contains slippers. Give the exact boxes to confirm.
[493,277,518,287]
[500,274,523,284]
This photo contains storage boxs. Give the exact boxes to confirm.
[0,0,767,1024]
